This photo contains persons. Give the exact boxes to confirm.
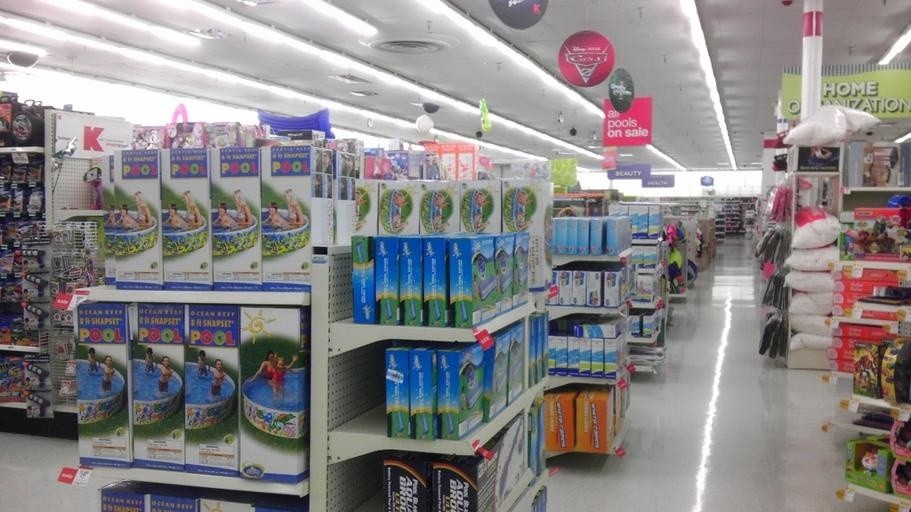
[103,190,305,233]
[102,356,116,391]
[272,355,299,401]
[87,346,100,372]
[159,357,174,392]
[211,360,225,394]
[248,352,276,388]
[198,351,208,374]
[356,189,534,230]
[146,347,155,375]
